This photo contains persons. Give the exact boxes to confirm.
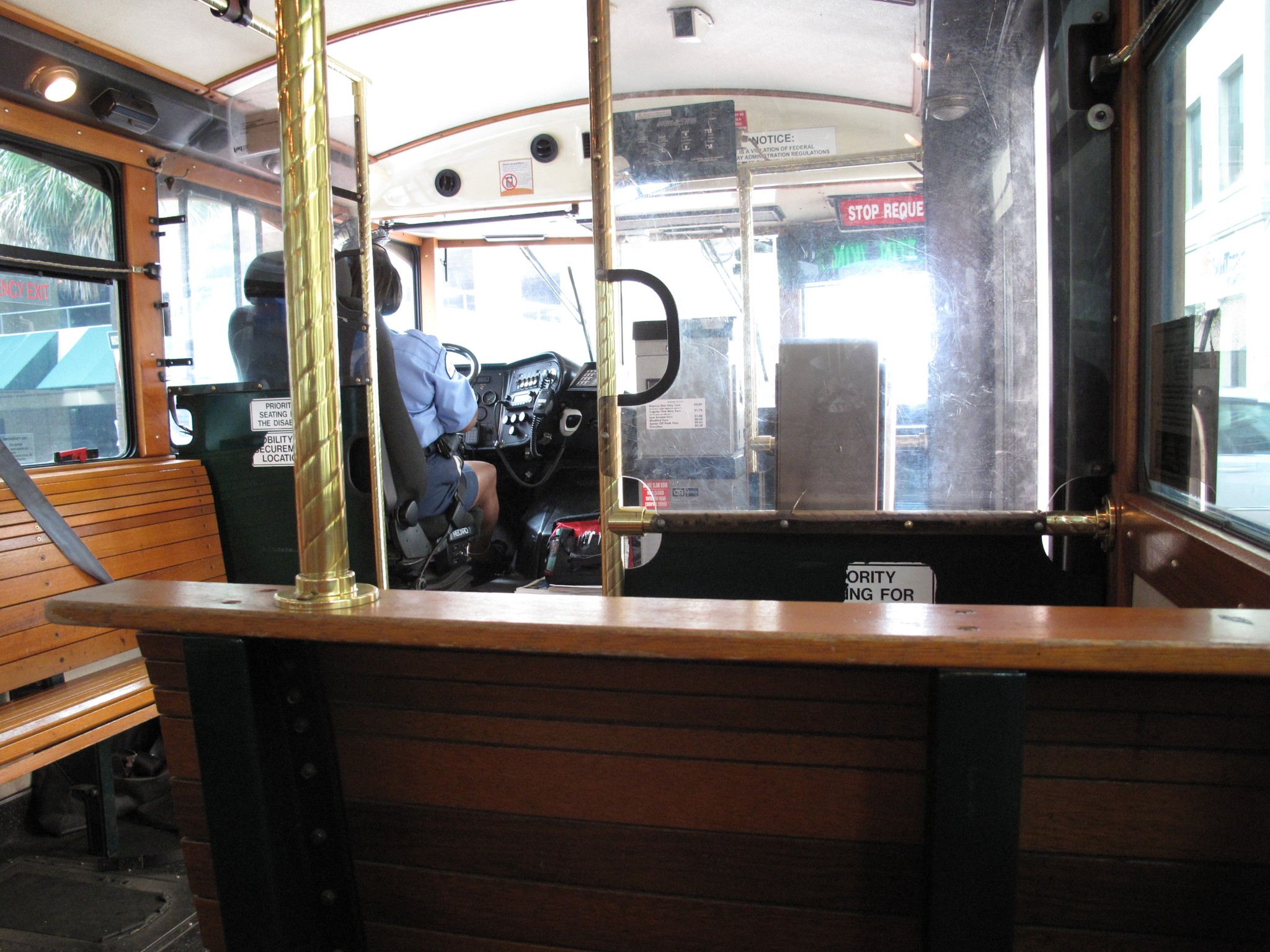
[341,240,521,584]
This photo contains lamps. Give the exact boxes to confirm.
[30,64,78,102]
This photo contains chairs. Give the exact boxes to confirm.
[228,249,484,591]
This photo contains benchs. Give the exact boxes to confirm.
[0,458,226,874]
[42,578,1270,952]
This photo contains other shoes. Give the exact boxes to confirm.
[469,539,515,584]
